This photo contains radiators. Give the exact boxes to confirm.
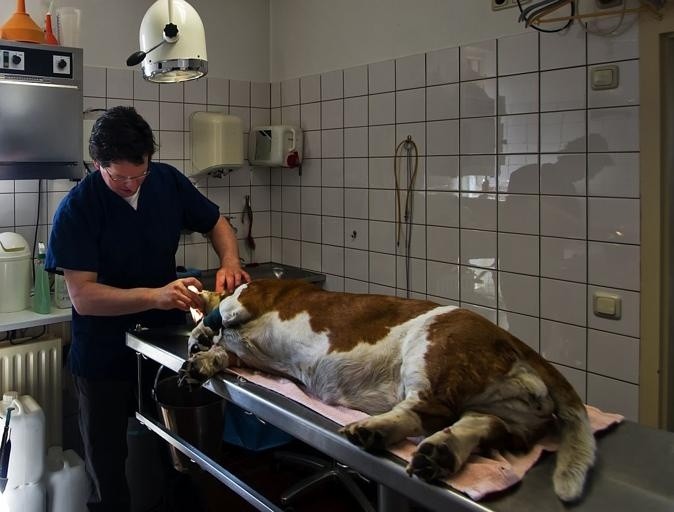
[0,332,64,450]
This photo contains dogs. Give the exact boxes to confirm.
[176,277,599,505]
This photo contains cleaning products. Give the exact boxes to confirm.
[33,240,51,314]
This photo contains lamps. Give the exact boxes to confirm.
[125,0,209,86]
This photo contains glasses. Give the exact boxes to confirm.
[100,160,152,182]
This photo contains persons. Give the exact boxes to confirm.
[45,105,252,512]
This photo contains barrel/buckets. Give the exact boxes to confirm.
[2,390,46,486]
[43,445,93,512]
[152,365,227,476]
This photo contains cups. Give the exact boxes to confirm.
[57,6,80,47]
